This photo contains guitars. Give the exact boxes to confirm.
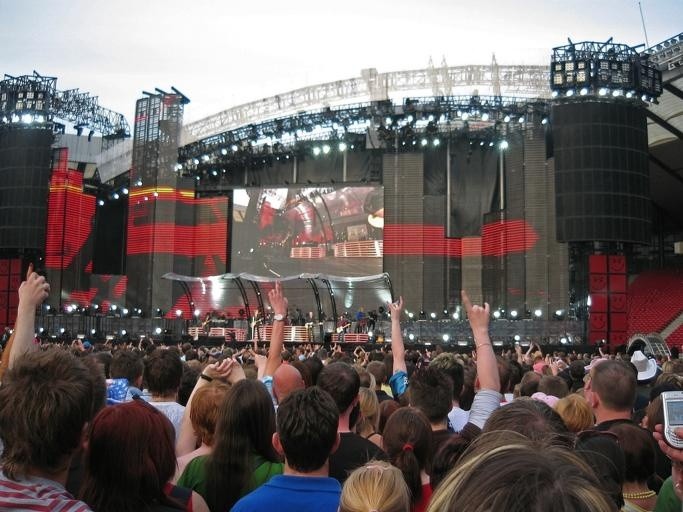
[305,322,323,330]
[336,323,351,334]
[250,317,264,327]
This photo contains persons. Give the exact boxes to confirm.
[258,186,384,249]
[1,262,683,512]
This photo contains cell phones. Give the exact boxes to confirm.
[660,391,683,450]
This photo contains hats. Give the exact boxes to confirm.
[630,350,657,381]
